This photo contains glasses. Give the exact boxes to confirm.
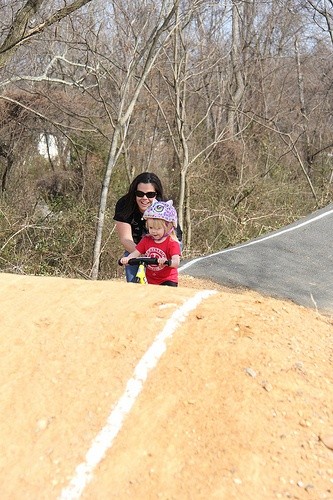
[135,190,157,198]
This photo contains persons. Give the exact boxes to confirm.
[116,199,181,288]
[112,172,183,282]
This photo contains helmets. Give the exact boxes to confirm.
[142,198,178,227]
